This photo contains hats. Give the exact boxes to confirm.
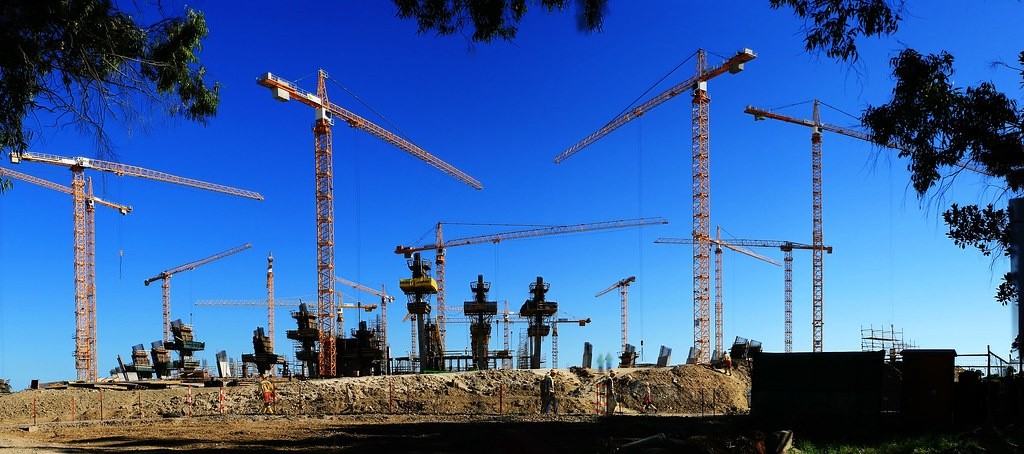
[644,382,649,386]
[611,370,616,375]
[550,368,557,374]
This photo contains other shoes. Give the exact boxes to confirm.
[655,409,659,414]
[723,372,727,374]
[729,373,732,376]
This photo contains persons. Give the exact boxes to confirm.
[597,353,613,370]
[606,370,617,414]
[540,368,560,415]
[258,374,275,415]
[641,382,658,414]
[722,351,732,376]
